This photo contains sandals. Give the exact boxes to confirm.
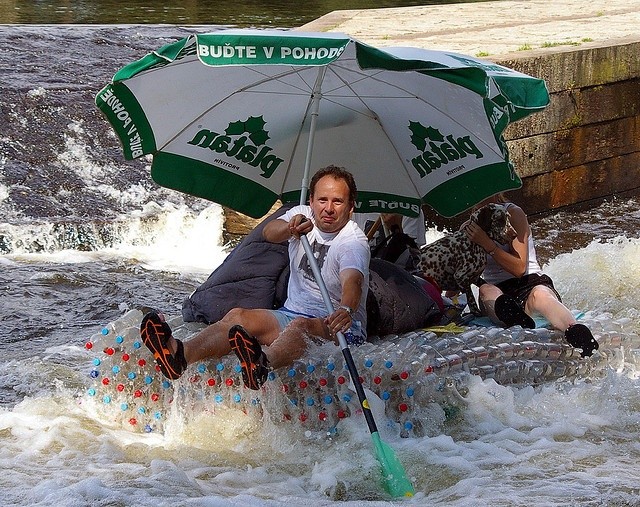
[228,323,270,391]
[494,292,536,329]
[140,309,188,380]
[564,324,599,357]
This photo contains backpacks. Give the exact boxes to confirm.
[371,224,422,272]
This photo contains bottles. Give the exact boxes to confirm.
[85,308,640,439]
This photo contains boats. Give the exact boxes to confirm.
[85,308,639,450]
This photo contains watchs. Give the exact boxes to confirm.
[338,305,355,319]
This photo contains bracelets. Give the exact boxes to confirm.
[489,246,498,256]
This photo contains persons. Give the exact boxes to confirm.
[444,192,599,358]
[140,165,372,390]
[350,208,426,258]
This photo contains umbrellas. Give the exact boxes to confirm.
[94,28,551,206]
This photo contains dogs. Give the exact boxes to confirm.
[404,202,518,318]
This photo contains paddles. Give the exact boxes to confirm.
[295,228,415,500]
